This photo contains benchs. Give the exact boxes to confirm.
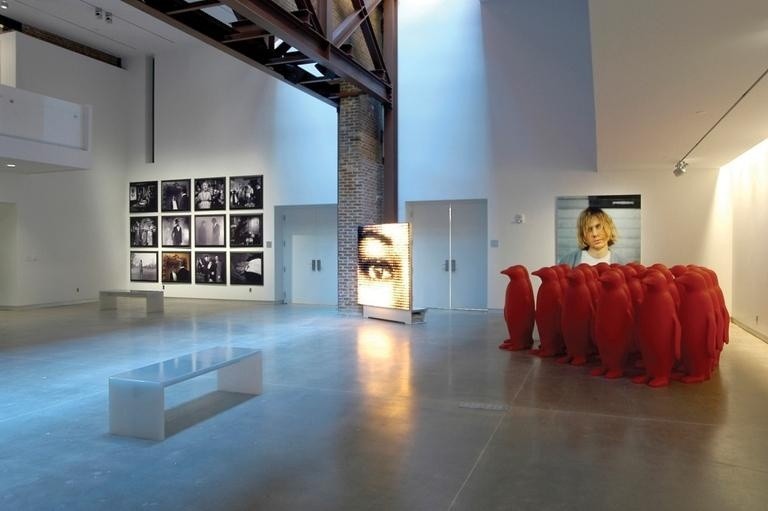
[106,346,265,441]
[98,289,163,314]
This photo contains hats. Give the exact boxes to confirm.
[175,219,180,224]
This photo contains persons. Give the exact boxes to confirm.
[558,207,627,270]
[358,223,411,310]
[129,174,263,283]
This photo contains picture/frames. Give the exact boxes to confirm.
[128,174,265,286]
